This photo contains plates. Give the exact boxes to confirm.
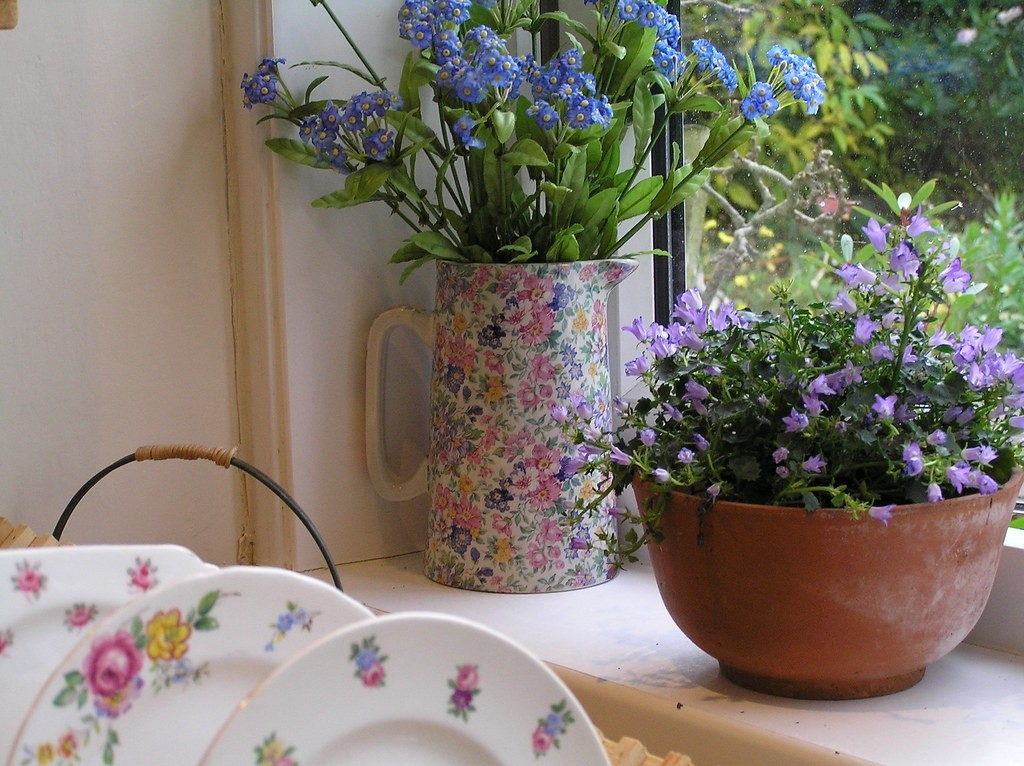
[201,611,610,766]
[0,545,219,766]
[9,568,374,766]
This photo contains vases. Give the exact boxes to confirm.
[630,464,1023,700]
[365,256,640,591]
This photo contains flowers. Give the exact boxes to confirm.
[239,0,830,267]
[549,205,1023,580]
